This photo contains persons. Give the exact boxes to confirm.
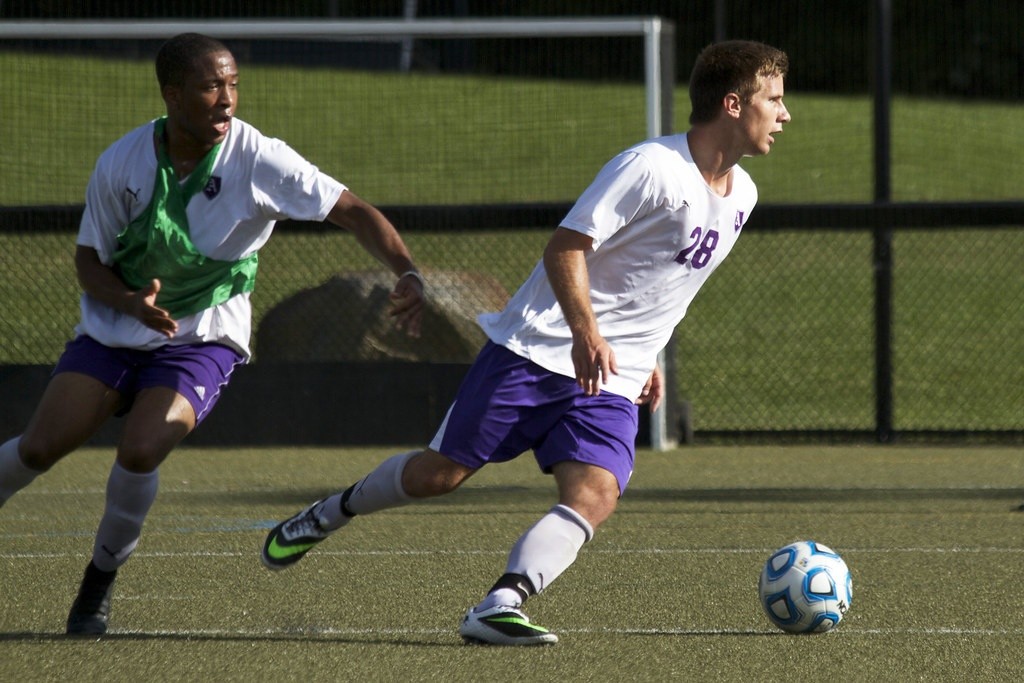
[264,39,791,646]
[1,32,426,637]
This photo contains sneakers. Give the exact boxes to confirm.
[67,588,111,637]
[261,499,336,571]
[458,604,561,645]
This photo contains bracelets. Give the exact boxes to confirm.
[399,271,424,289]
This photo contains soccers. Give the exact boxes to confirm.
[756,538,855,637]
